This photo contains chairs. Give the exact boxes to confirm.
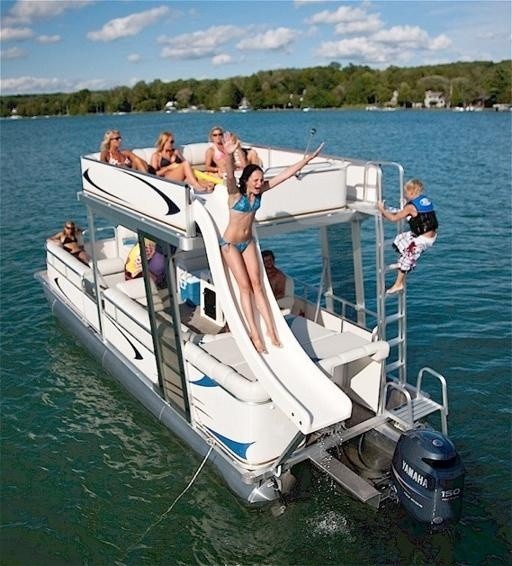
[269,271,295,315]
[95,142,216,185]
[58,224,165,315]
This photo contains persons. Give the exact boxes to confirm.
[261,250,286,300]
[99,130,149,173]
[205,126,259,173]
[51,221,90,264]
[151,132,214,192]
[222,131,324,352]
[378,179,438,293]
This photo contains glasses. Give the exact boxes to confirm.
[66,226,75,230]
[111,136,121,140]
[170,140,175,144]
[212,133,222,136]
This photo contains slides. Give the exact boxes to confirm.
[194,199,353,436]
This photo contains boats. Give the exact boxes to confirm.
[33,142,467,532]
